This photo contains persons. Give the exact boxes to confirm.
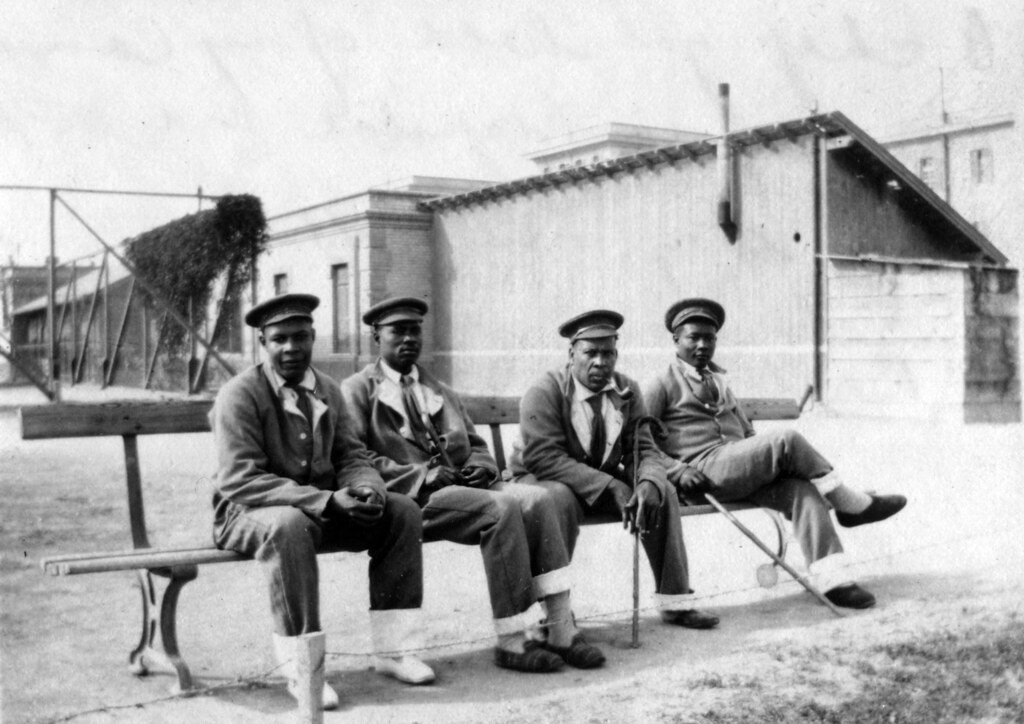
[507,310,717,630]
[642,299,906,610]
[339,297,606,673]
[209,293,436,708]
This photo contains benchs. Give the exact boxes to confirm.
[15,395,876,700]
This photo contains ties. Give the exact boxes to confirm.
[402,375,430,454]
[586,395,606,463]
[294,387,312,424]
[700,370,720,402]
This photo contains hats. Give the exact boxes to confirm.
[362,297,429,327]
[245,295,319,330]
[559,310,622,344]
[665,299,725,333]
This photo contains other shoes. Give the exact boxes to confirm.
[375,655,436,683]
[494,642,564,672]
[288,679,339,710]
[545,633,606,668]
[826,584,875,608]
[837,494,907,527]
[662,611,720,628]
